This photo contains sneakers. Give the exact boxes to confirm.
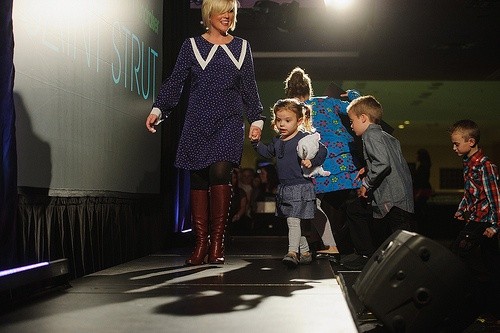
[282,254,298,266]
[300,253,312,263]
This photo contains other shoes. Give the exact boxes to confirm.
[317,251,339,254]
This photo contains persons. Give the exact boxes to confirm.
[283,68,363,267]
[448,120,500,255]
[250,99,328,266]
[346,96,415,249]
[146,0,265,266]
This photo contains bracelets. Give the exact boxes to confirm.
[361,178,369,189]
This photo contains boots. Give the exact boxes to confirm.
[207,185,233,263]
[185,190,209,265]
